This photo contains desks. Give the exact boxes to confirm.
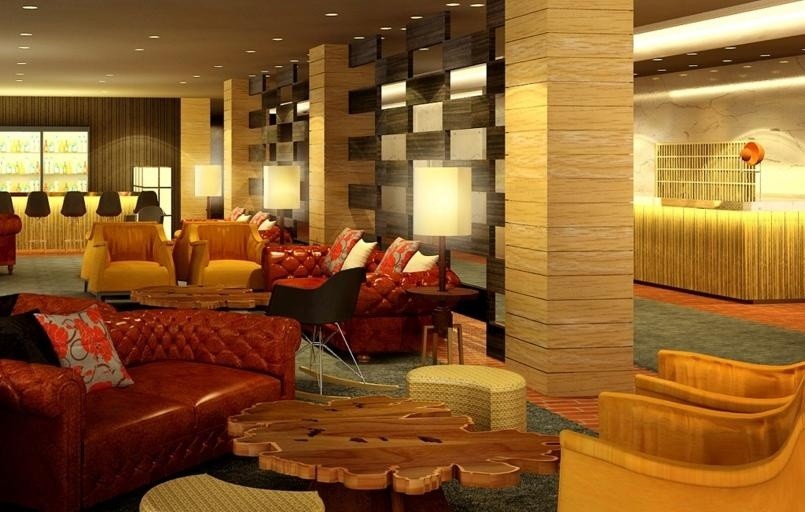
[130,285,272,310]
[227,392,561,511]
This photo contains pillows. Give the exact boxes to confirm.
[321,227,366,277]
[258,219,277,231]
[249,211,270,226]
[231,207,247,221]
[375,237,422,272]
[33,303,134,393]
[340,238,378,272]
[402,250,440,272]
[236,214,252,222]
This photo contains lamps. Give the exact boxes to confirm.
[263,166,301,244]
[194,164,222,220]
[405,167,479,364]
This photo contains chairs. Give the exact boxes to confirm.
[25,190,160,254]
[558,376,805,512]
[636,348,805,413]
[267,267,399,404]
[80,218,268,302]
[0,191,23,276]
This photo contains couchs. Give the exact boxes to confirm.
[1,293,117,317]
[1,308,302,512]
[263,242,461,364]
[248,219,293,245]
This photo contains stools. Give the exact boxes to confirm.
[405,364,528,432]
[139,473,325,512]
